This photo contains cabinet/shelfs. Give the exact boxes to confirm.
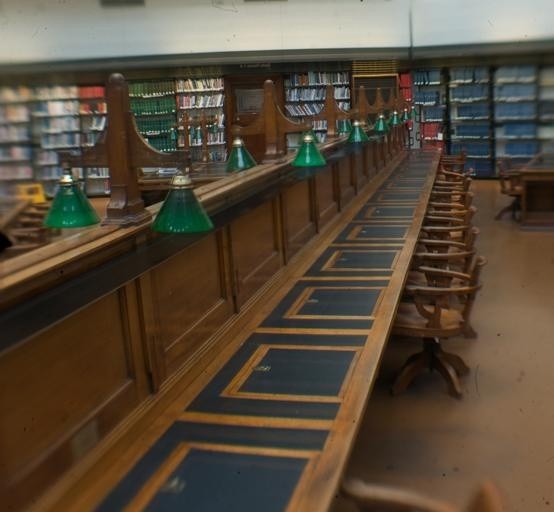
[412,64,538,179]
[1,68,356,192]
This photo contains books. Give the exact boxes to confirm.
[399,66,554,177]
[126,77,229,179]
[2,85,111,197]
[283,72,351,147]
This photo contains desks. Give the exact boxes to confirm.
[40,144,443,509]
[521,151,553,226]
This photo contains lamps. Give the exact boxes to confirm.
[41,171,213,238]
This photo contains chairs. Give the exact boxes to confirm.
[492,154,523,223]
[381,149,488,400]
